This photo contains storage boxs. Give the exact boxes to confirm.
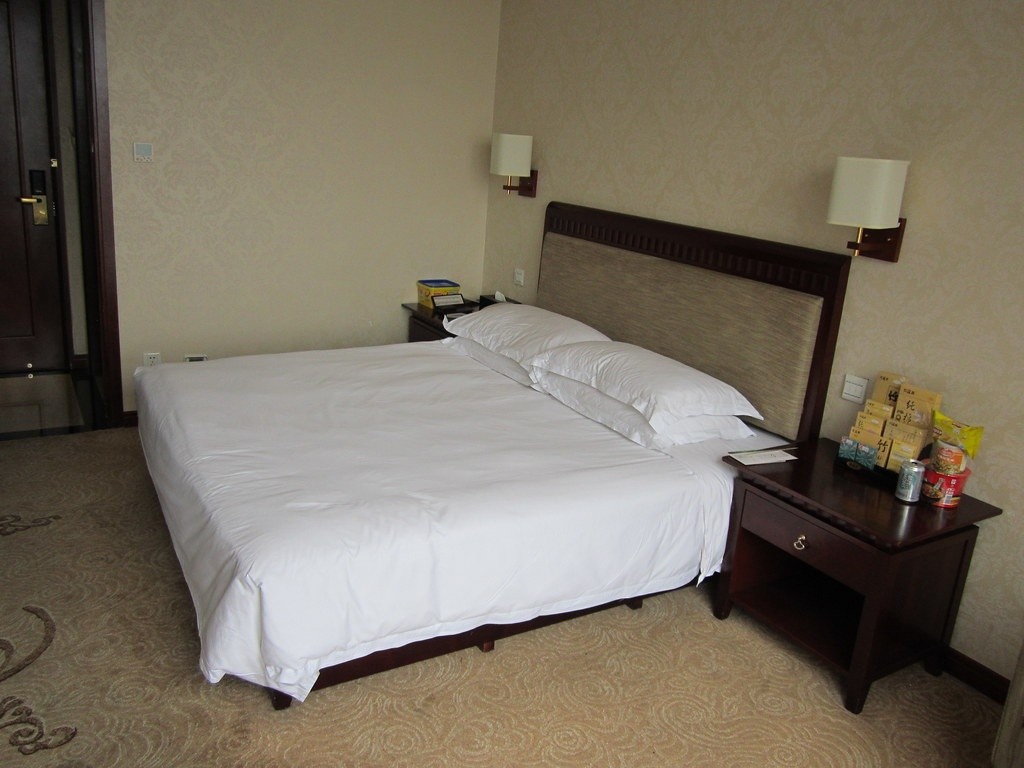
[416,278,460,309]
[839,373,940,475]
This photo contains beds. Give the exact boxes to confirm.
[134,201,854,710]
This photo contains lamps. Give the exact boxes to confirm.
[826,157,910,262]
[490,133,538,198]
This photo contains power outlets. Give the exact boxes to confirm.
[143,353,160,367]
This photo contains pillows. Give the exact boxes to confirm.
[443,335,550,395]
[441,302,612,383]
[523,340,764,435]
[532,365,758,450]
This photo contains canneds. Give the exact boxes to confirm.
[895,459,925,502]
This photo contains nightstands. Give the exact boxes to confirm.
[716,436,1002,713]
[400,298,480,342]
